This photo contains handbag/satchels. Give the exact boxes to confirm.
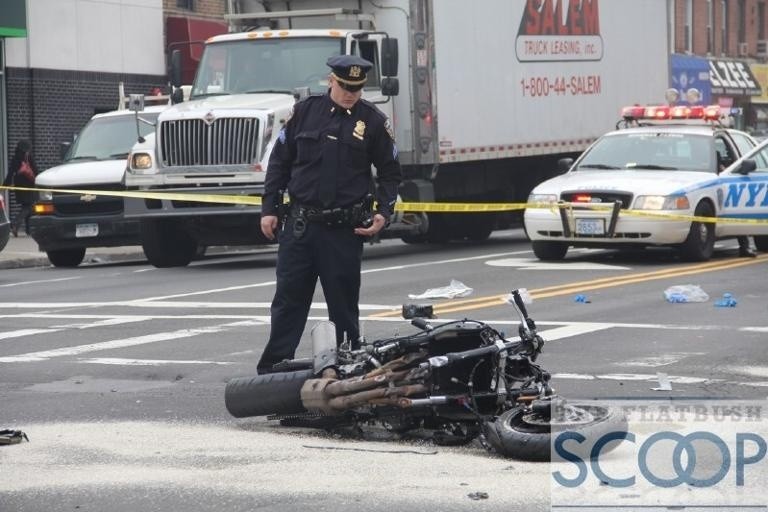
[17,152,35,183]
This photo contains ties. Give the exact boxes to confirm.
[318,107,344,207]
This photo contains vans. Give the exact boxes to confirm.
[28,95,174,268]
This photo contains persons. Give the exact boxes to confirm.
[255,54,402,376]
[2,141,37,236]
[693,140,756,257]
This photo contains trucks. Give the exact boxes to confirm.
[122,0,668,268]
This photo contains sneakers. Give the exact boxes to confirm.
[12,224,18,237]
[740,246,758,257]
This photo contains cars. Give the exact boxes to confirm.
[524,88,768,261]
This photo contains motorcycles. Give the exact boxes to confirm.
[225,289,628,462]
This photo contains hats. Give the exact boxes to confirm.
[326,55,374,92]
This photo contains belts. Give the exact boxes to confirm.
[287,208,353,225]
